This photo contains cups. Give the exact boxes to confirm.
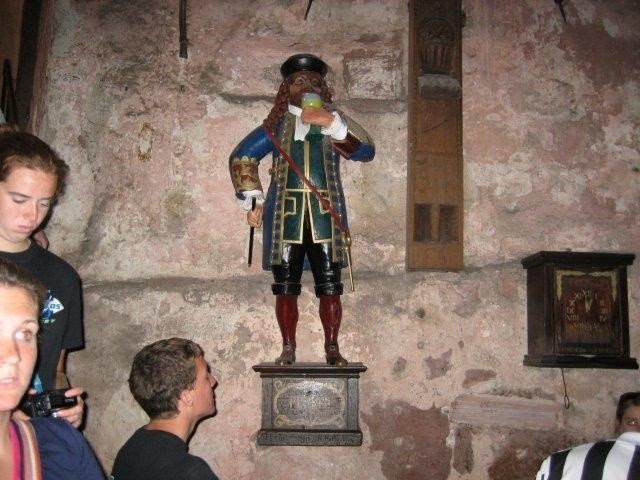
[299,93,323,109]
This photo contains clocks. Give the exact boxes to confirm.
[555,272,619,350]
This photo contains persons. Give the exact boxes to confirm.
[612,391,640,442]
[0,258,110,479]
[227,52,377,366]
[108,336,221,479]
[0,132,87,432]
[0,110,51,252]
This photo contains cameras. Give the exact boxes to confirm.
[20,387,78,418]
